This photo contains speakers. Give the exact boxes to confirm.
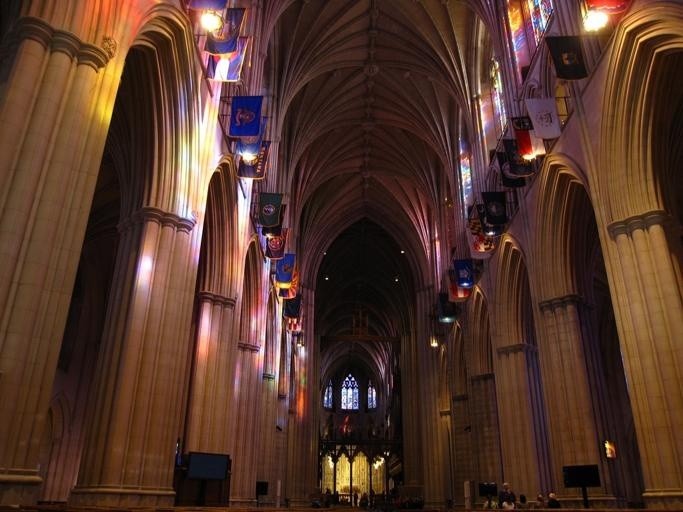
[479,482,498,497]
[563,464,601,488]
[173,452,231,507]
[256,480,268,495]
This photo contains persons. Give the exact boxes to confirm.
[484,483,560,510]
[354,490,358,507]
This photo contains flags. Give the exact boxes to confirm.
[496,152,527,187]
[511,116,547,161]
[236,116,267,154]
[544,36,588,79]
[191,1,250,82]
[470,191,508,255]
[447,259,475,301]
[229,96,264,136]
[438,293,457,323]
[258,193,284,227]
[265,228,288,258]
[503,138,536,174]
[237,141,271,178]
[276,253,296,282]
[525,96,560,140]
[277,272,302,318]
[262,204,287,237]
